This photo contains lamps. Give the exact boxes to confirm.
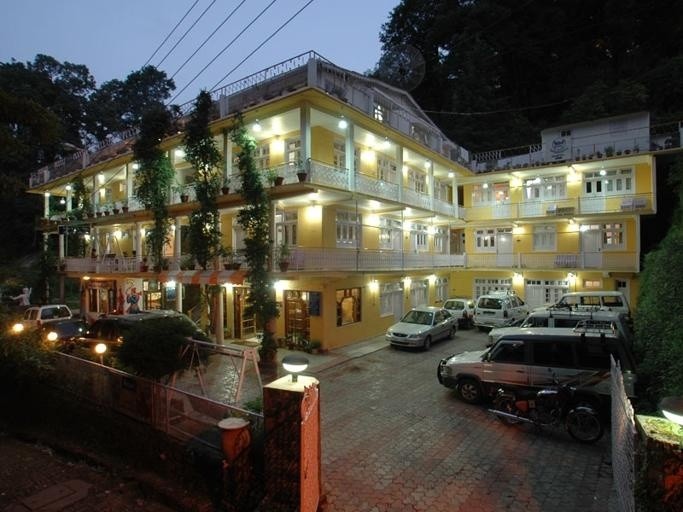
[657,392,683,430]
[94,342,107,365]
[281,355,310,384]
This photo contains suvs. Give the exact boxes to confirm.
[384,288,644,419]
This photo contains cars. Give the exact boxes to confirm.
[1,302,210,373]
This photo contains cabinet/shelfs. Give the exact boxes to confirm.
[236,295,257,339]
[143,288,166,311]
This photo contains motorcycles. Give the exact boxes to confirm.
[489,366,607,445]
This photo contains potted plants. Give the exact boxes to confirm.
[295,158,307,183]
[221,178,231,195]
[576,145,645,161]
[53,259,67,271]
[46,197,152,229]
[139,245,291,273]
[266,170,284,186]
[174,184,190,203]
